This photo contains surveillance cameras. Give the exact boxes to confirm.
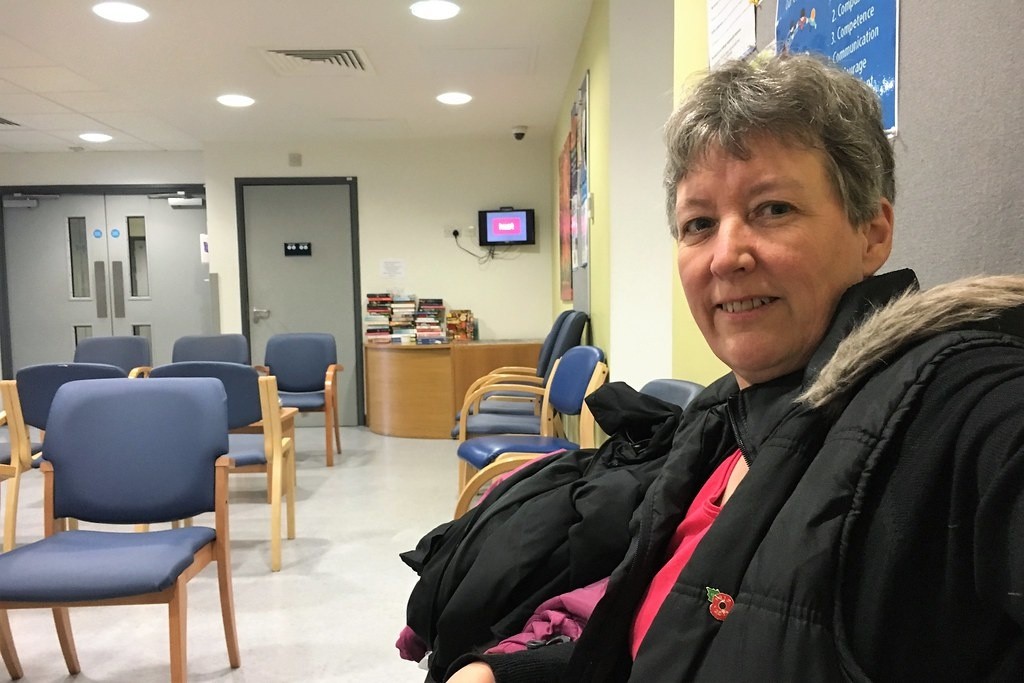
[512,125,527,140]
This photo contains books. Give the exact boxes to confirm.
[415,298,448,344]
[389,298,417,346]
[365,292,392,345]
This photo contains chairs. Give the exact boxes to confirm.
[171,333,252,366]
[0,379,241,683]
[253,332,344,468]
[72,336,154,380]
[126,362,296,573]
[0,362,127,558]
[452,310,709,524]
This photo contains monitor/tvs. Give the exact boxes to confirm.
[479,208,535,245]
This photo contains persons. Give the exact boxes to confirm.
[446,46,1024,683]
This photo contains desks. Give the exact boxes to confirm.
[363,341,542,438]
